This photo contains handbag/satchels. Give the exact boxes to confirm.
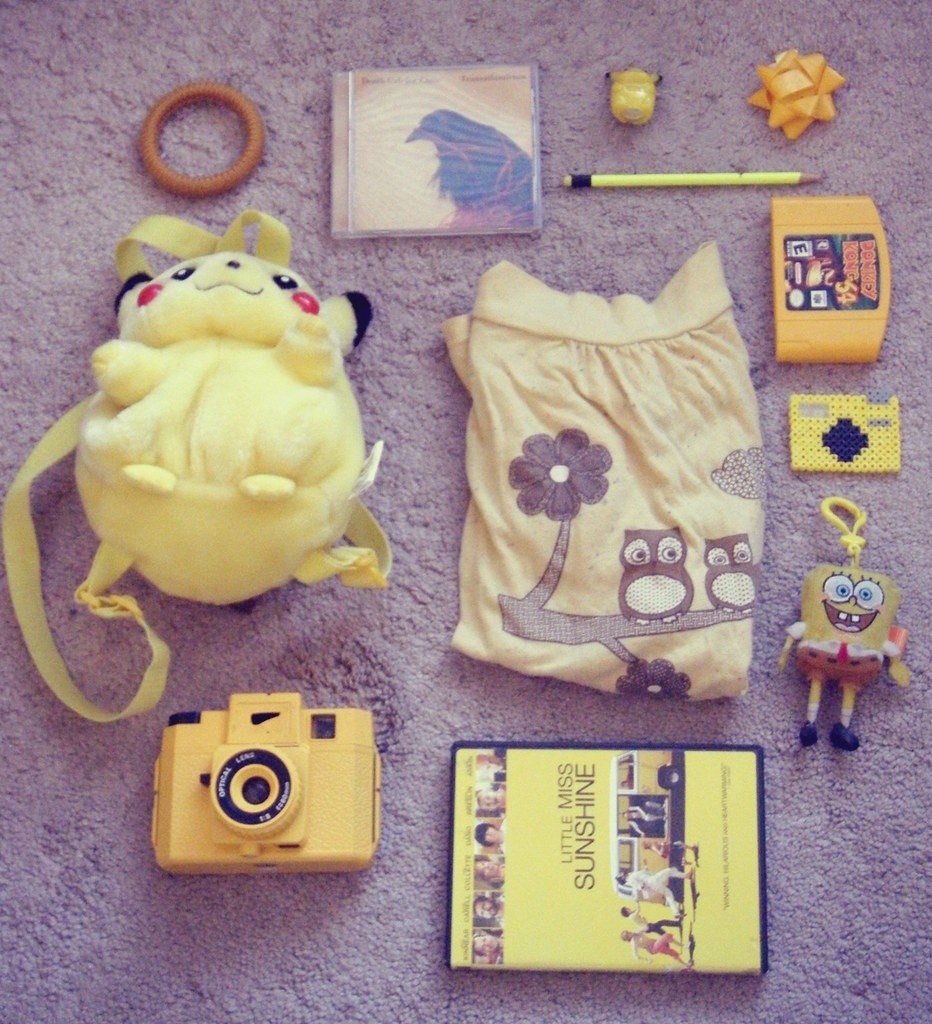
[1,212,393,725]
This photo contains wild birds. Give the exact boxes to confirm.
[405,108,534,229]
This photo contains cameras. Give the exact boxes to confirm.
[151,692,383,877]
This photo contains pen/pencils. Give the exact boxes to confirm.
[563,172,821,189]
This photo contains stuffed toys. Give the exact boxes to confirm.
[779,565,911,751]
[74,250,372,607]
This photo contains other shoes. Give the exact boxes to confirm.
[693,846,699,860]
[673,913,687,917]
[688,866,694,880]
